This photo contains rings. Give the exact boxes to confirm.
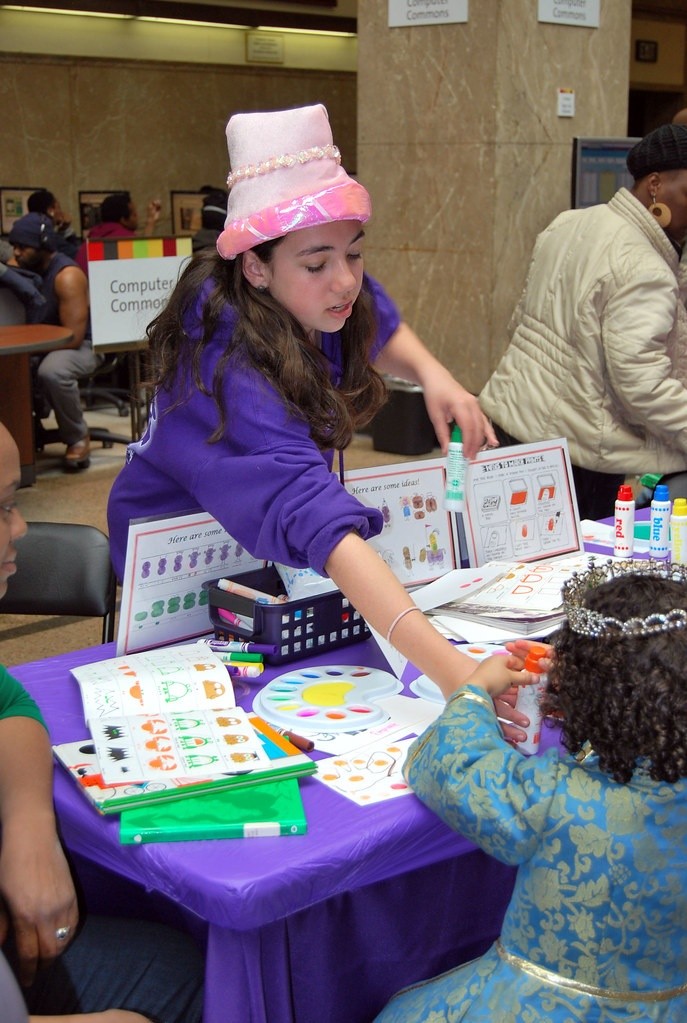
[56,926,71,940]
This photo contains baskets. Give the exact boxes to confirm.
[202,566,372,665]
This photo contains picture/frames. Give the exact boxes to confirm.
[76,189,123,237]
[0,186,46,238]
[169,189,212,235]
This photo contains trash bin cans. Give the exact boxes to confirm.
[371,377,435,455]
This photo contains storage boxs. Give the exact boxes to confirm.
[200,568,372,670]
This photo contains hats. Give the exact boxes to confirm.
[202,186,230,229]
[626,122,687,179]
[7,212,55,253]
[216,103,372,259]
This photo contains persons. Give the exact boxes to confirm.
[478,111,687,522]
[0,189,161,467]
[106,105,529,750]
[373,574,687,1023]
[193,194,227,253]
[0,421,152,1023]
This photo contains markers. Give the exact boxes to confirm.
[218,608,253,631]
[197,639,279,655]
[218,578,301,617]
[233,667,260,677]
[223,662,265,673]
[213,651,264,663]
[264,720,315,753]
[278,594,289,601]
[225,665,238,674]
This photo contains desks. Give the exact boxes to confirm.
[5,503,687,1023]
[0,325,76,491]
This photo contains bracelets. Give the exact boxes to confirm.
[387,607,421,642]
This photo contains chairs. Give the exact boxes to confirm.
[0,521,118,645]
[36,354,131,454]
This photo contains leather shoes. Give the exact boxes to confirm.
[64,433,89,462]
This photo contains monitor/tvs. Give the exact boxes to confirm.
[572,137,642,210]
